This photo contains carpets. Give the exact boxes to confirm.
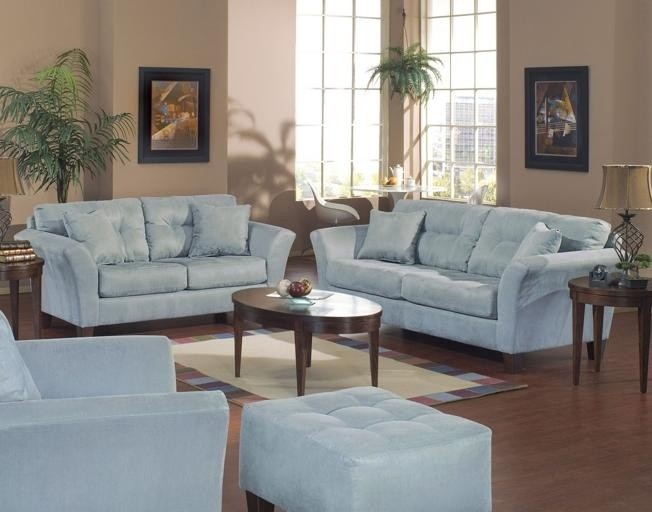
[167,320,533,412]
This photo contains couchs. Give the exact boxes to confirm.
[240,383,493,510]
[0,305,230,510]
[11,193,296,337]
[310,199,629,370]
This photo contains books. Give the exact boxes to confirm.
[0,254,36,263]
[0,240,32,249]
[0,248,34,255]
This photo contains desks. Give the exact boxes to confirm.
[344,181,431,209]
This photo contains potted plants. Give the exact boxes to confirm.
[615,252,652,291]
[366,38,445,109]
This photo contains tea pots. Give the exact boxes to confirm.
[389,164,407,186]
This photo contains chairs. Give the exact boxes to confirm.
[297,177,362,262]
[463,184,491,208]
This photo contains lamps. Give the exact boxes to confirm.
[594,164,652,293]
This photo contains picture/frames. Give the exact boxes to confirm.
[138,66,210,163]
[524,66,589,171]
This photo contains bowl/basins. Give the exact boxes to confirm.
[287,298,315,311]
